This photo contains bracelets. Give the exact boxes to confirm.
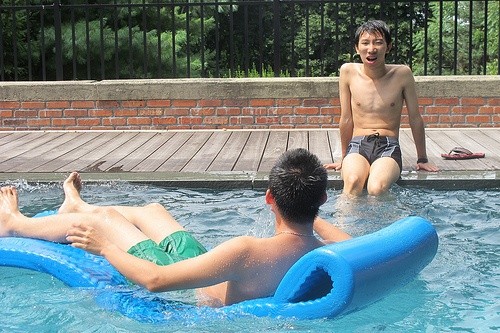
[417,158,428,164]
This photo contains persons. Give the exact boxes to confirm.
[322,19,440,231]
[0,149,355,309]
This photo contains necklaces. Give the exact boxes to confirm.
[274,232,316,237]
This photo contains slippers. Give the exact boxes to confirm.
[441,147,485,159]
